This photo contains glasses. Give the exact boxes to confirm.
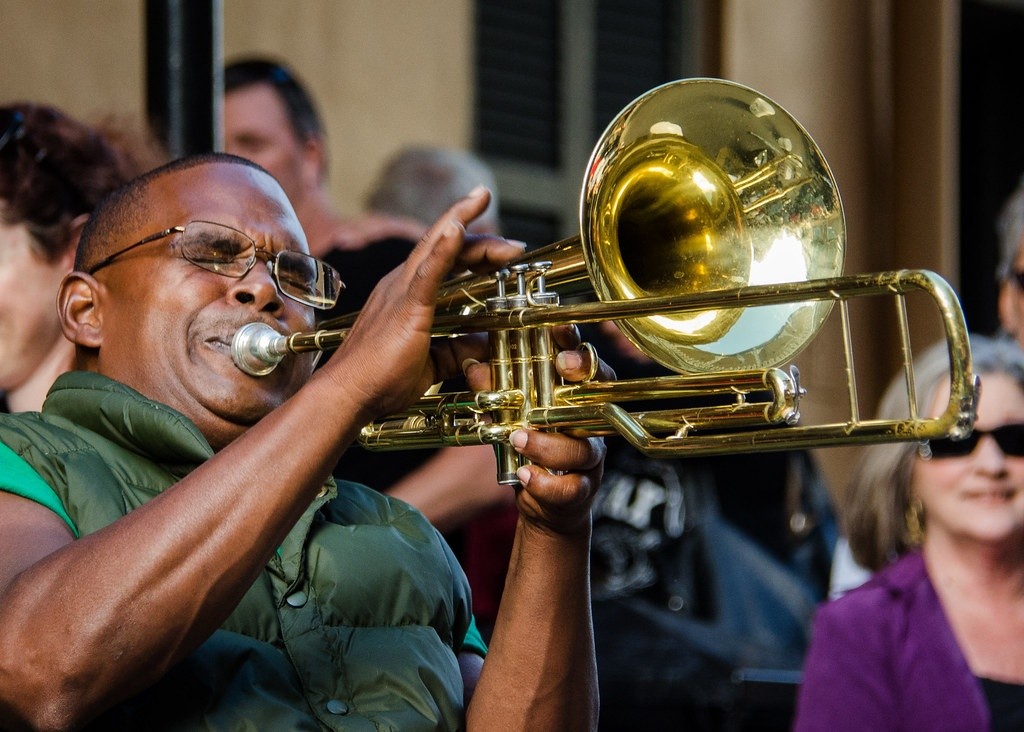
[924,422,1024,458]
[89,218,348,311]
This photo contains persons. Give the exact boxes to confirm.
[1,58,1024,731]
[0,154,618,732]
[792,332,1024,731]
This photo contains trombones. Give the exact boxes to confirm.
[231,75,980,487]
